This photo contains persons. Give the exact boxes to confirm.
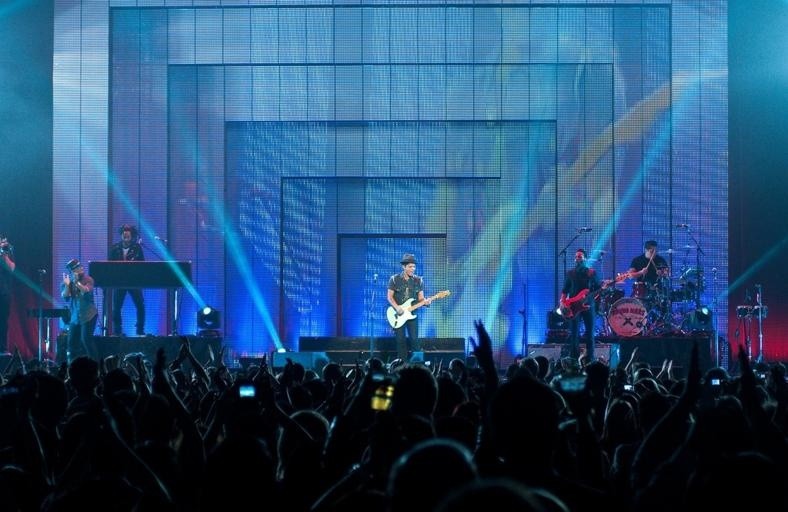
[108,223,146,337]
[0,317,788,512]
[0,231,17,353]
[387,253,432,362]
[627,240,670,336]
[559,249,613,364]
[58,258,99,383]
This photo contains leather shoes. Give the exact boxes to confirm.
[136,330,146,337]
[0,350,12,357]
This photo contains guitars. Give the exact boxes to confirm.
[560,274,627,320]
[387,290,451,328]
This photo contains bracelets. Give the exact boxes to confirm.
[75,282,80,287]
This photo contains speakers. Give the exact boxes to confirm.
[527,343,621,379]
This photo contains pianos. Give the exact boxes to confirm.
[88,260,192,288]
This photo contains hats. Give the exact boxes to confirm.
[400,254,418,264]
[66,259,80,272]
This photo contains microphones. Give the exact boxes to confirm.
[373,274,379,280]
[676,224,687,228]
[40,269,47,274]
[712,268,717,273]
[135,237,143,245]
[154,236,168,243]
[579,226,592,232]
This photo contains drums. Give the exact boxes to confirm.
[632,283,653,299]
[607,296,647,336]
[670,287,691,302]
